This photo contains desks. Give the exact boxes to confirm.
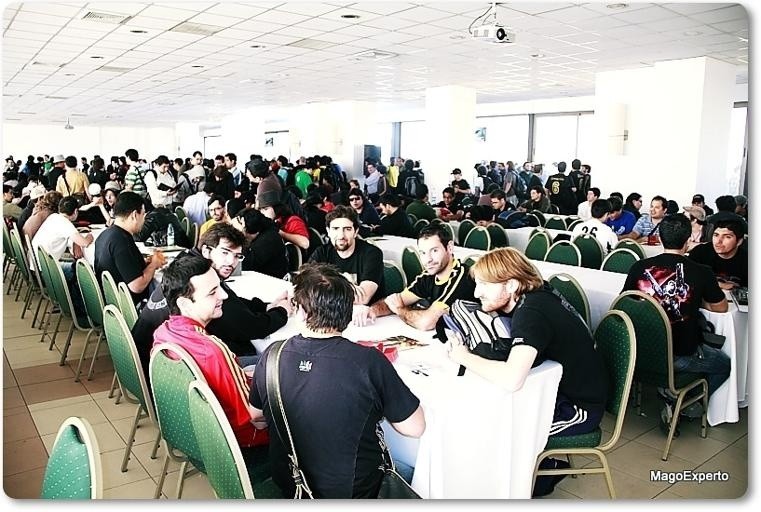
[158,269,564,501]
[69,223,191,285]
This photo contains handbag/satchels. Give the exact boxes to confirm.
[498,211,530,229]
[379,466,423,499]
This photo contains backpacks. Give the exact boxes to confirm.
[480,175,492,195]
[405,176,420,198]
[435,300,543,369]
[512,169,528,199]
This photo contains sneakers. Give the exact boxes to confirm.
[537,460,572,497]
[658,399,704,440]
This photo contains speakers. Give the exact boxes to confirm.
[364,144,381,175]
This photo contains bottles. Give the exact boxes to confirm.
[167,223,175,246]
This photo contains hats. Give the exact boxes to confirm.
[683,205,705,222]
[31,185,50,200]
[106,180,120,192]
[256,191,280,209]
[53,154,66,163]
[692,195,704,202]
[450,169,461,174]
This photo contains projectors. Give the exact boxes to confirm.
[65,125,74,129]
[472,25,514,43]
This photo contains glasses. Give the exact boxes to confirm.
[208,244,245,261]
[349,197,360,201]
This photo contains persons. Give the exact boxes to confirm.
[93,191,167,311]
[3,148,747,307]
[248,259,427,499]
[131,222,296,415]
[149,250,270,471]
[619,214,730,441]
[686,219,748,290]
[354,222,481,331]
[444,246,609,499]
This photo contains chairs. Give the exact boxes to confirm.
[547,276,593,331]
[611,289,710,461]
[42,417,103,500]
[2,218,138,380]
[368,202,649,289]
[383,260,408,296]
[283,242,303,272]
[189,380,291,498]
[149,343,211,498]
[101,305,161,473]
[531,307,636,498]
[169,207,200,251]
